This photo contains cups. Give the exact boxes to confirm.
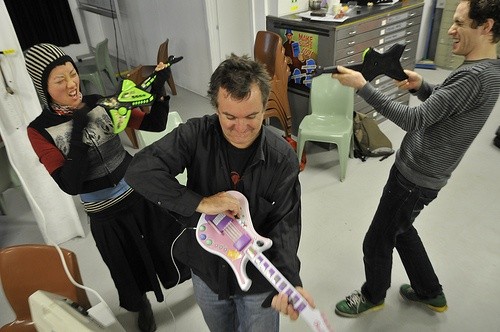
[332,3,342,17]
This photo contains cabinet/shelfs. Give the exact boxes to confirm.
[267,0,425,126]
[428,0,500,70]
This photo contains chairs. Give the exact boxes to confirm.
[75,38,183,151]
[254,28,293,139]
[0,244,91,332]
[298,72,357,181]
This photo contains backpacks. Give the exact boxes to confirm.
[354,111,395,162]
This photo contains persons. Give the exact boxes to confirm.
[124,54,315,332]
[25,43,192,332]
[331,0,500,317]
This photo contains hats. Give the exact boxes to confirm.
[285,29,293,35]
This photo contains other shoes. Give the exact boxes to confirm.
[138,301,156,332]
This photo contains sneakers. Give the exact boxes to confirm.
[335,289,385,318]
[399,284,448,313]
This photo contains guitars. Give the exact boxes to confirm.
[306,42,410,83]
[97,55,184,134]
[195,191,335,332]
